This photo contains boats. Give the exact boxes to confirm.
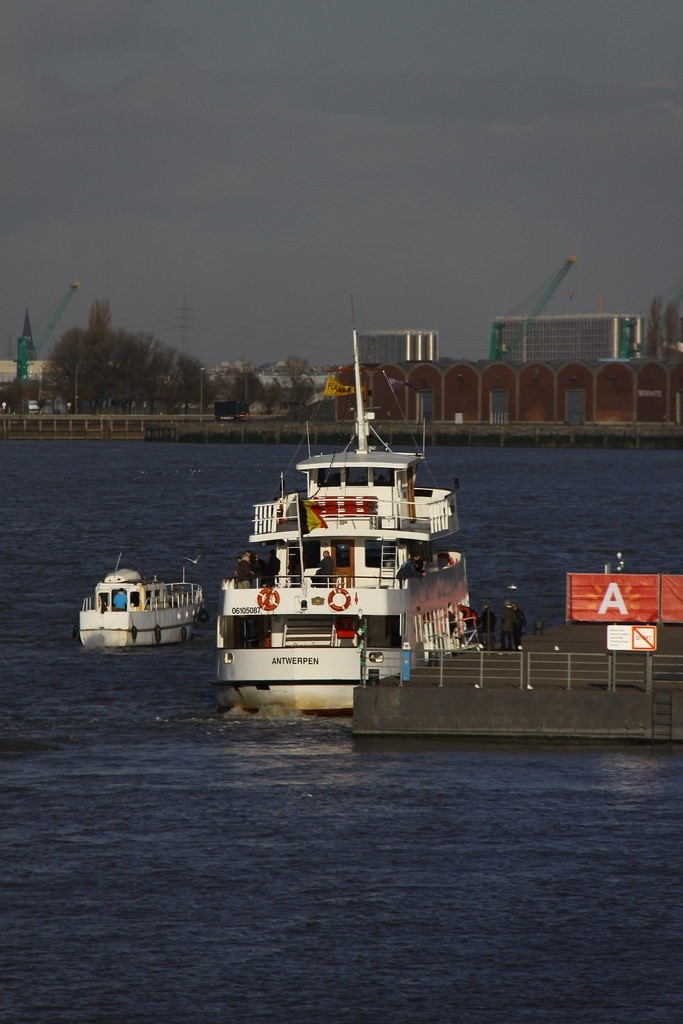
[72,552,211,650]
[208,330,497,716]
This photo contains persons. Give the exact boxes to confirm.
[397,552,427,590]
[458,604,478,644]
[235,549,280,589]
[114,588,128,611]
[318,551,334,587]
[482,606,497,645]
[500,600,527,650]
[294,551,307,586]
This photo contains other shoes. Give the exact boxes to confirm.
[499,647,515,652]
[517,646,523,651]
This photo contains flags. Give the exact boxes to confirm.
[299,499,328,534]
[386,373,418,392]
[323,374,355,396]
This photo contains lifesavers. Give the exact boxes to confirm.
[436,552,454,567]
[198,609,210,622]
[328,588,351,612]
[257,588,281,611]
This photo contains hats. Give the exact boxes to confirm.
[512,600,518,604]
[503,600,511,605]
